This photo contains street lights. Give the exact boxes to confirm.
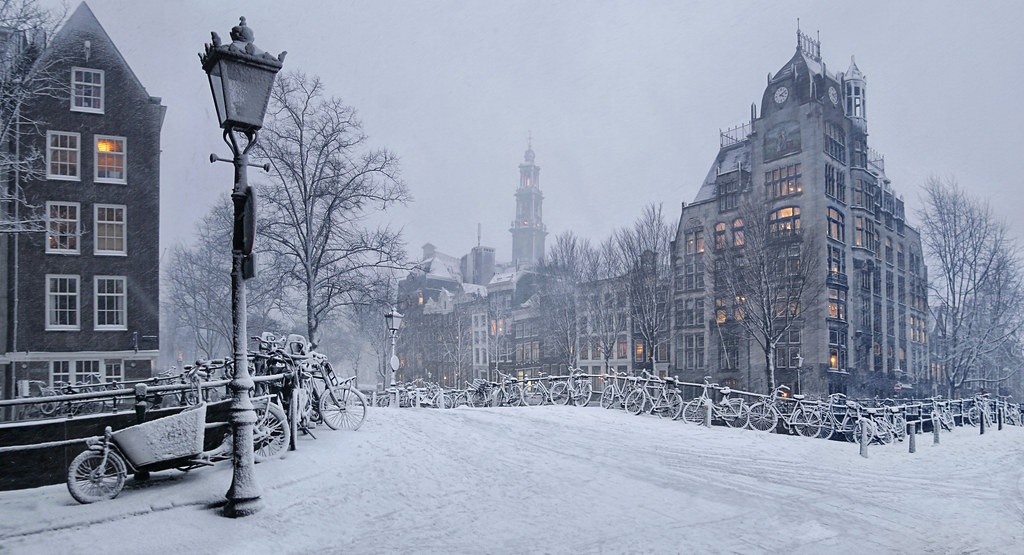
[384,306,405,408]
[199,16,288,517]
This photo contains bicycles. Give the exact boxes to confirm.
[371,351,1022,446]
[3,330,369,506]
